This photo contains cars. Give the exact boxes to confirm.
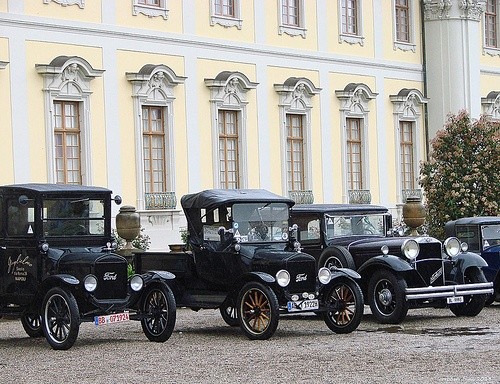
[130,188,365,341]
[443,216,500,306]
[0,183,178,351]
[286,202,494,325]
